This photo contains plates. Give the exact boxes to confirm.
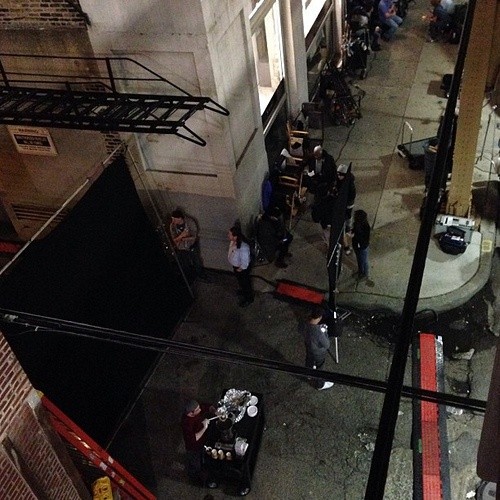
[246,395,258,418]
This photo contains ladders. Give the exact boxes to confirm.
[34,389,157,500]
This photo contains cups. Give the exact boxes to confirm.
[206,447,232,461]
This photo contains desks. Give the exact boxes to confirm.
[209,388,266,482]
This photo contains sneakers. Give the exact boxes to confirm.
[317,381,335,390]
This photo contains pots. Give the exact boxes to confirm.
[215,419,234,432]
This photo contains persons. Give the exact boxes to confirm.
[361,5,381,50]
[352,209,371,277]
[169,211,207,285]
[359,41,370,80]
[182,399,214,482]
[294,145,355,227]
[419,139,439,220]
[378,0,402,42]
[228,226,253,306]
[303,306,335,391]
[258,209,293,268]
[427,0,451,42]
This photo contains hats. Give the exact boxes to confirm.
[337,164,348,173]
[184,399,198,412]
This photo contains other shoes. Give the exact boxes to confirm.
[237,289,244,296]
[358,275,368,282]
[239,300,252,307]
[352,272,358,278]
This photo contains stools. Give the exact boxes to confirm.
[186,448,206,473]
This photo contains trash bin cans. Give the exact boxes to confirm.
[423,139,452,193]
